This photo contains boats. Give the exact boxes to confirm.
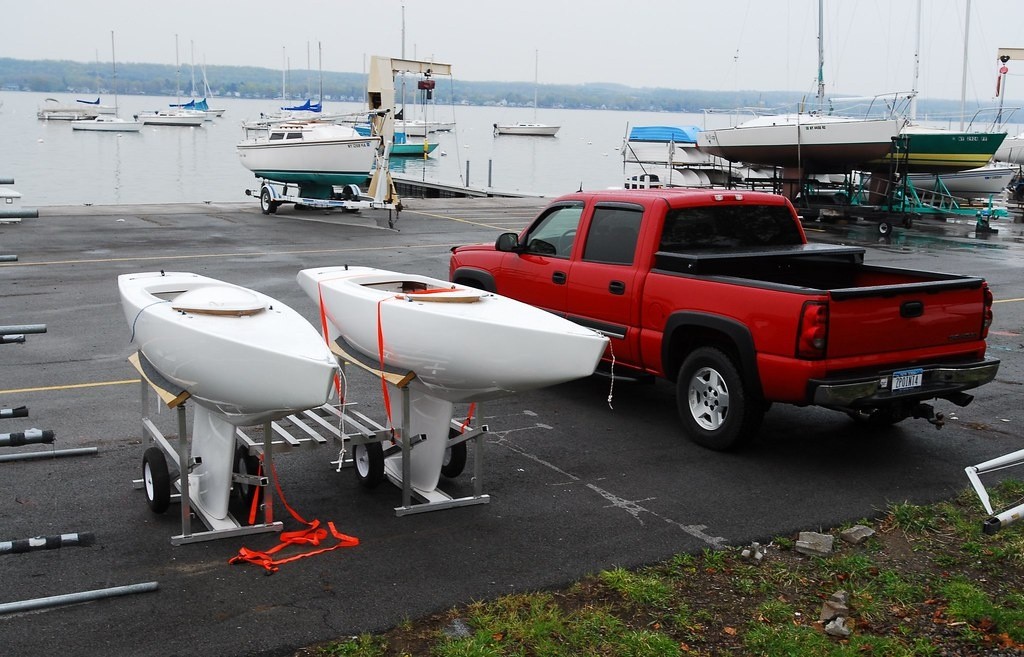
[237,120,381,185]
[300,265,609,401]
[623,0,1024,200]
[117,269,340,426]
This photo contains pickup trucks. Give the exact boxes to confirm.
[447,185,1001,453]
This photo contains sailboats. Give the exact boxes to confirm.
[245,41,456,156]
[175,38,226,122]
[132,33,207,126]
[35,31,146,133]
[493,49,561,136]
